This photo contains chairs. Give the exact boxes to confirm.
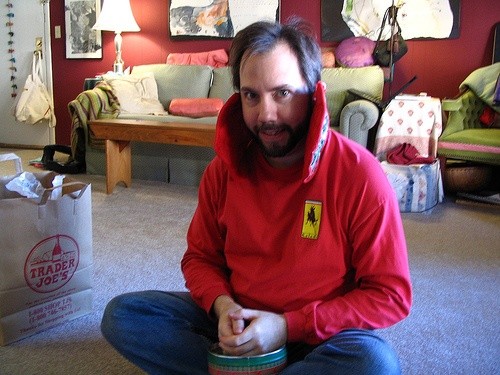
[437,64,500,205]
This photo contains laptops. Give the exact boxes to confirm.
[347,76,417,108]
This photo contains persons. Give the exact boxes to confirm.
[99,15,412,375]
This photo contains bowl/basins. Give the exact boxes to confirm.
[207,342,288,375]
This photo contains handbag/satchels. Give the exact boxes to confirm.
[1,152,95,348]
[11,50,57,128]
[370,5,408,67]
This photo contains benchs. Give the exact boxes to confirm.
[84,64,384,196]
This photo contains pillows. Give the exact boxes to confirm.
[107,70,168,118]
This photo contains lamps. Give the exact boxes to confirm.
[92,1,140,78]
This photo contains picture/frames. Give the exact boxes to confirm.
[64,0,102,59]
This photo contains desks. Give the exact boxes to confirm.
[87,119,216,195]
[373,97,442,162]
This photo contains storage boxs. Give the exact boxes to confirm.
[381,158,444,213]
